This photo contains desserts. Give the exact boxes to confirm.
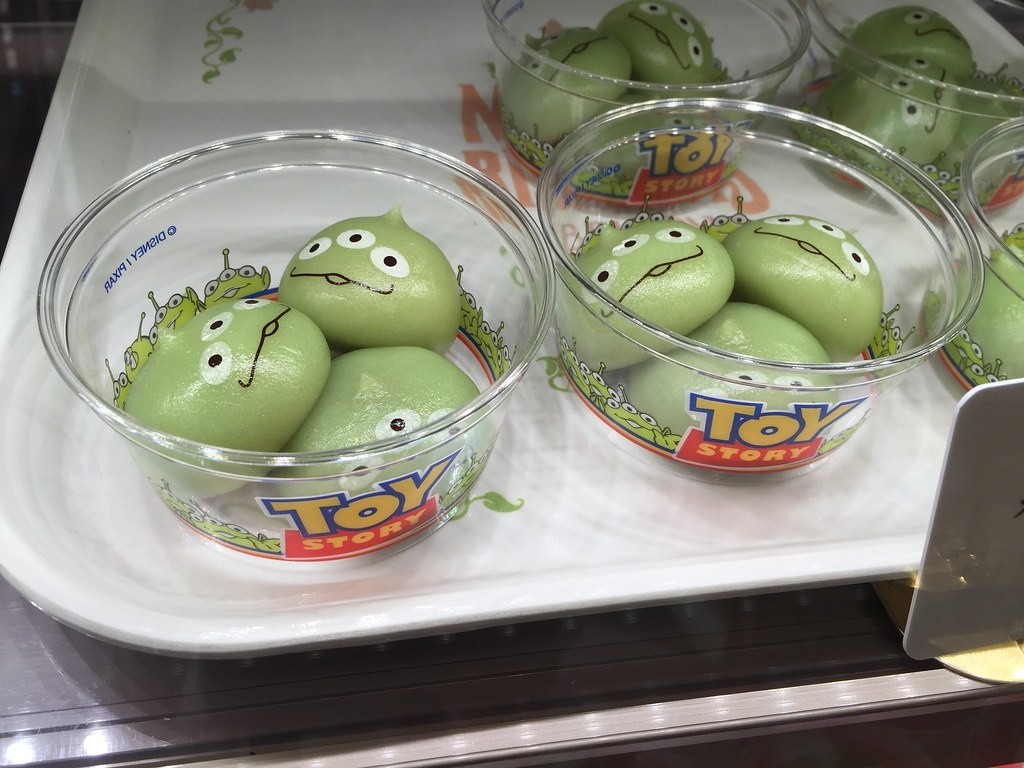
[120,0,1024,521]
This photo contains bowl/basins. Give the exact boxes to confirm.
[38,131,558,565]
[920,116,1024,393]
[478,0,812,220]
[537,96,986,484]
[791,0,1024,219]
